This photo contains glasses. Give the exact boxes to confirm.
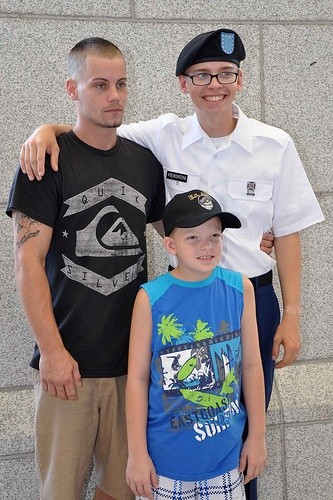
[184,72,239,87]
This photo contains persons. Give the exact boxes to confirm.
[125,189,268,500]
[19,28,326,500]
[6,37,275,500]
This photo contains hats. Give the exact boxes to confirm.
[175,28,247,78]
[161,189,241,237]
